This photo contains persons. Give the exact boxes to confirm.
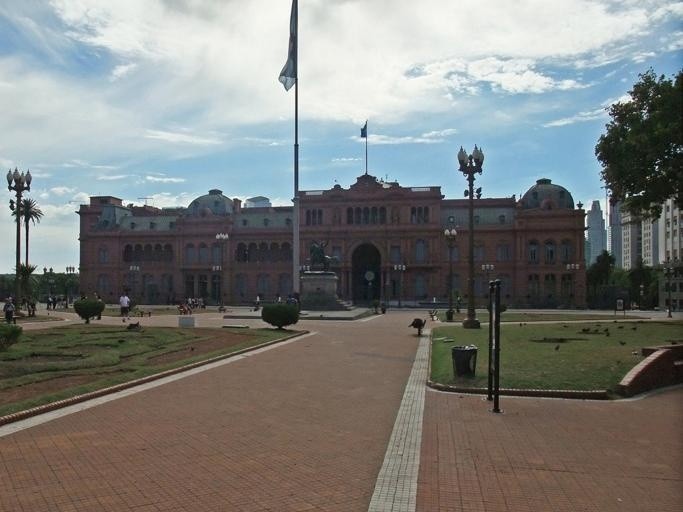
[118,290,131,321]
[178,296,204,316]
[2,294,68,323]
[272,292,298,304]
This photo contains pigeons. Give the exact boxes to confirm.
[619,340,626,345]
[519,323,522,326]
[127,317,130,320]
[563,325,569,328]
[190,345,194,351]
[555,344,560,350]
[523,323,526,325]
[121,318,125,322]
[581,320,637,337]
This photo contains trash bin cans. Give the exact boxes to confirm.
[446,311,453,320]
[452,346,478,377]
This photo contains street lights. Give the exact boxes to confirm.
[663,267,675,318]
[129,266,139,295]
[459,144,484,319]
[567,264,580,308]
[444,228,458,320]
[481,265,495,282]
[394,263,406,308]
[212,232,228,305]
[299,264,310,275]
[7,167,32,312]
[65,266,75,305]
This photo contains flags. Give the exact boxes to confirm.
[360,121,366,138]
[279,1,294,91]
